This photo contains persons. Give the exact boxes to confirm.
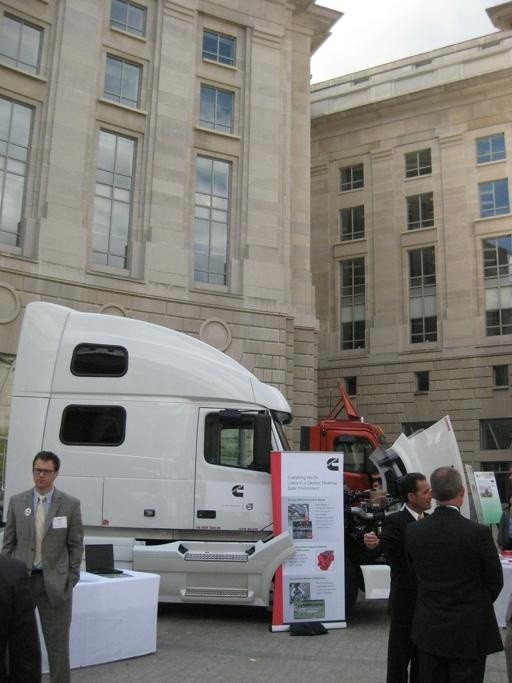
[399,466,504,683]
[0,450,84,683]
[370,477,383,492]
[362,473,435,683]
[0,554,43,683]
[495,493,512,550]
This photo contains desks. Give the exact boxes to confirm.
[3,568,162,678]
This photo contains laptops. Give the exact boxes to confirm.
[85,544,123,574]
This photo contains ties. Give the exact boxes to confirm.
[33,494,47,572]
